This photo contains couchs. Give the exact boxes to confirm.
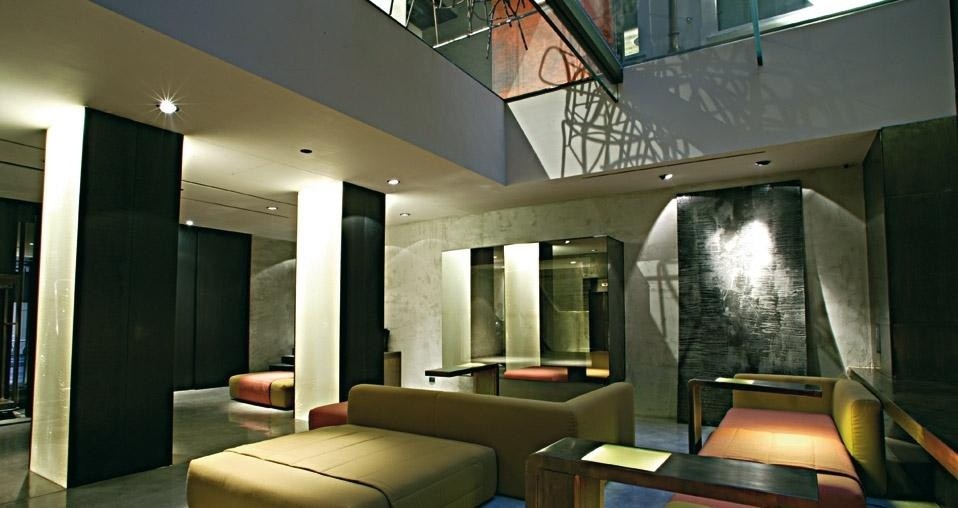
[307,378,636,502]
[522,371,892,508]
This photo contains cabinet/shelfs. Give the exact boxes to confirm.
[438,233,626,371]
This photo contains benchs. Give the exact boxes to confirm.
[229,363,295,409]
[184,422,499,508]
